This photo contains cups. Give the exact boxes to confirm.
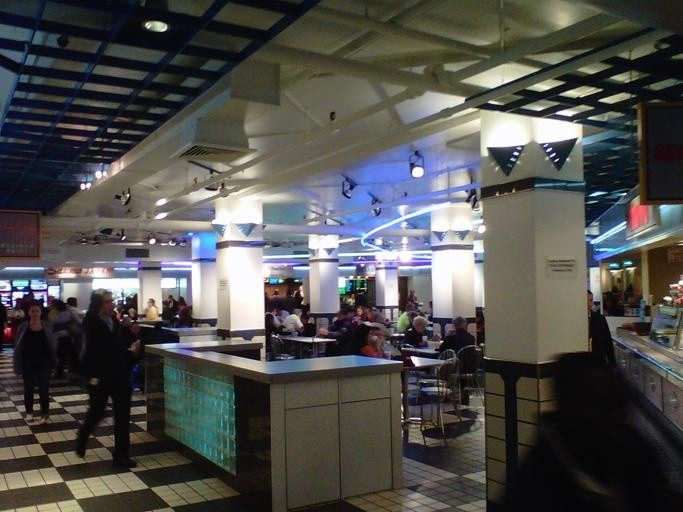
[422,336,427,346]
[291,331,297,337]
[644,304,651,316]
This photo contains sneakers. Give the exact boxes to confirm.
[111,455,137,467]
[75,436,87,459]
[40,416,47,425]
[26,413,34,422]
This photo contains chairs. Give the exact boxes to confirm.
[269,321,484,448]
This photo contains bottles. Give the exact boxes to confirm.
[639,299,645,316]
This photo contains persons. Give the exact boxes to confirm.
[73,288,140,467]
[265,291,389,359]
[12,304,51,425]
[1,291,192,385]
[498,352,681,511]
[398,291,485,406]
[587,291,618,366]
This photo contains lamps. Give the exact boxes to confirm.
[138,1,176,38]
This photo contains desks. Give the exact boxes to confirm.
[142,338,407,510]
